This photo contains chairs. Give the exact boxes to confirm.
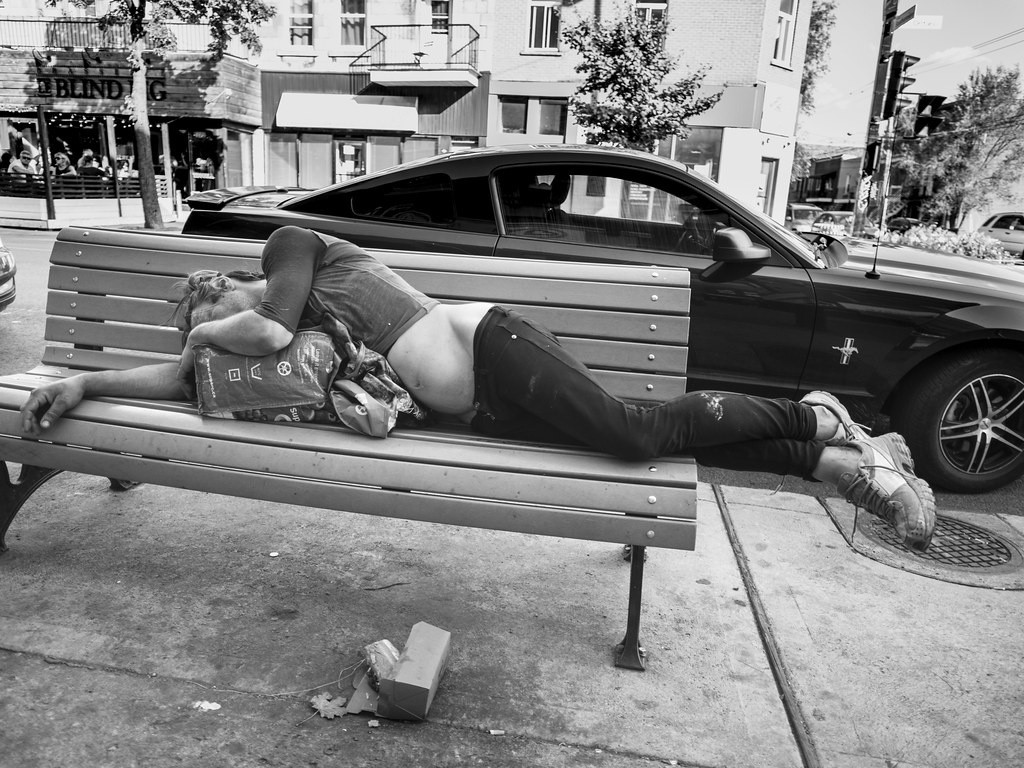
[546,174,574,224]
[517,182,552,227]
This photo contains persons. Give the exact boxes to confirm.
[0,153,12,169]
[78,156,105,180]
[37,156,50,175]
[17,224,938,550]
[78,147,93,167]
[116,160,131,181]
[7,150,35,183]
[50,152,76,179]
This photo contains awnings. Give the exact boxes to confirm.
[276,91,418,134]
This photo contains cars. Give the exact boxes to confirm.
[1,237,17,312]
[811,210,880,239]
[887,216,929,234]
[182,143,1024,493]
[978,211,1024,257]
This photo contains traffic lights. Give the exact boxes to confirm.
[883,53,921,121]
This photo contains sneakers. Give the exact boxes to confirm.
[798,390,873,446]
[836,431,937,553]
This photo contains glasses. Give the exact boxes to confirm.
[54,157,63,160]
[21,155,31,161]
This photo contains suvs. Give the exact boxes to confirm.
[783,202,825,232]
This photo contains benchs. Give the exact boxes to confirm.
[0,171,172,200]
[1,224,699,673]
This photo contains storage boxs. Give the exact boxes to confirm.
[344,619,452,721]
